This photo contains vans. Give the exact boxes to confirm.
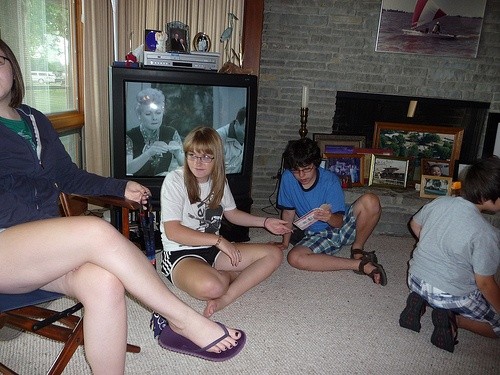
[31,71,56,84]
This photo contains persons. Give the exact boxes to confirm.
[0,38,246,375]
[125,88,246,176]
[160,126,293,318]
[171,33,207,52]
[399,157,500,354]
[429,165,441,175]
[268,138,387,286]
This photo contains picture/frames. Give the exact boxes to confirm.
[165,21,210,55]
[483,112,500,158]
[314,122,464,198]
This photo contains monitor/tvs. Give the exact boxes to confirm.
[106,67,259,201]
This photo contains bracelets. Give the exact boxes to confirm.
[215,235,223,246]
[264,217,269,227]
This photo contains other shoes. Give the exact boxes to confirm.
[431,308,459,353]
[399,292,428,333]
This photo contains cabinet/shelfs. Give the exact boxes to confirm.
[108,199,252,251]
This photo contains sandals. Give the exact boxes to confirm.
[359,250,388,286]
[351,245,367,276]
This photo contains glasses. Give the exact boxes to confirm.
[0,55,10,66]
[289,165,314,174]
[185,153,215,163]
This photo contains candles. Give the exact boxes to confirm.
[407,100,417,117]
[302,86,309,108]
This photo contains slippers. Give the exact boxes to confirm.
[158,322,246,362]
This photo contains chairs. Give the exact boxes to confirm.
[0,194,141,375]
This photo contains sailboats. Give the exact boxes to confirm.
[401,0,458,39]
